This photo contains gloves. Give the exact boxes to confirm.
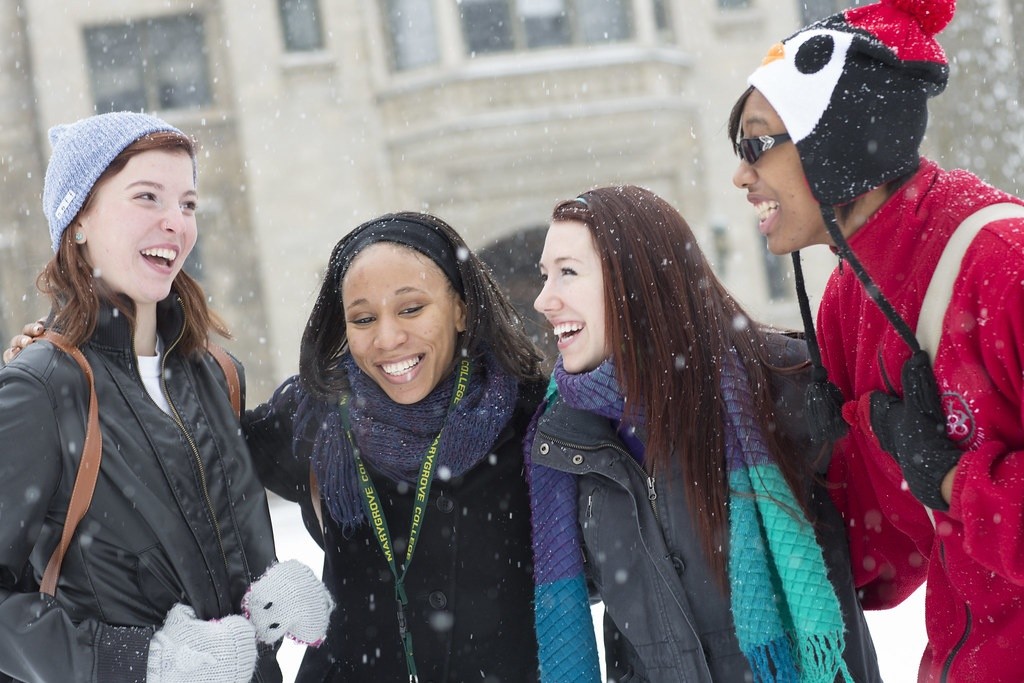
[856,361,963,511]
[241,559,336,646]
[145,602,258,683]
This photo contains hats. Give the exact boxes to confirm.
[44,111,196,257]
[749,0,955,442]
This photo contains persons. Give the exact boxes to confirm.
[728,1,1024,683]
[525,186,883,683]
[0,109,336,683]
[3,211,550,683]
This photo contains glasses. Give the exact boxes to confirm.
[735,133,789,165]
